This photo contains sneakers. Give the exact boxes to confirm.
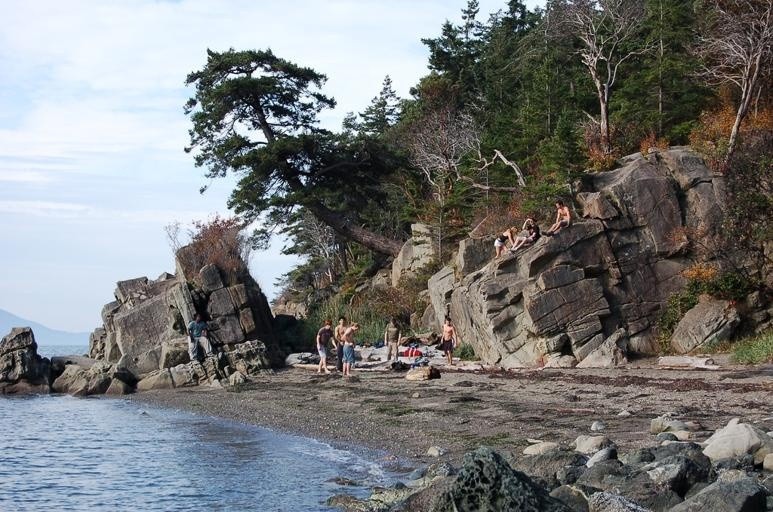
[509,230,553,253]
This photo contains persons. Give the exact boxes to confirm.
[439,317,458,366]
[187,313,214,364]
[335,315,346,372]
[383,319,402,360]
[509,217,539,252]
[493,226,518,258]
[340,321,359,374]
[406,338,419,358]
[541,200,572,238]
[315,320,337,374]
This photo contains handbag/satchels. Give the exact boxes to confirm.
[404,345,422,357]
